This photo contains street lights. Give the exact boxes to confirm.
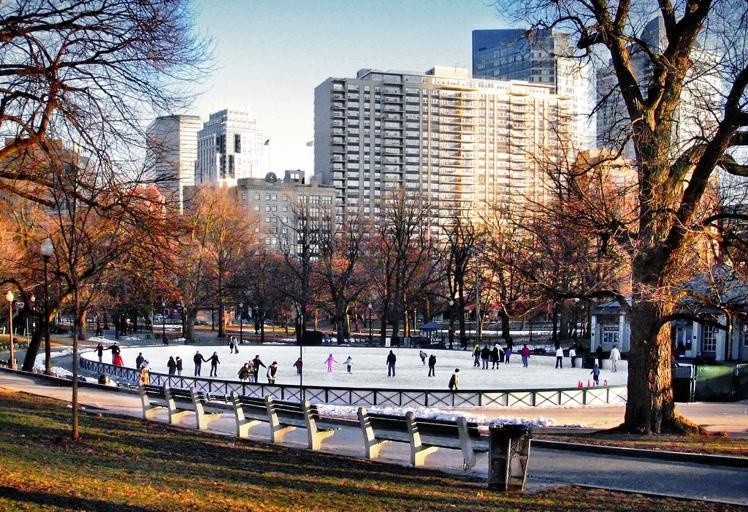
[237,299,247,344]
[447,299,455,348]
[39,243,55,375]
[6,289,18,371]
[368,297,374,339]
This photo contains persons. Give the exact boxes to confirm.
[293,357,303,375]
[206,350,221,377]
[595,345,603,369]
[323,332,329,345]
[248,360,256,382]
[140,359,151,371]
[193,350,206,377]
[94,343,106,362]
[471,337,513,370]
[167,356,176,378]
[231,336,239,354]
[448,368,460,390]
[324,353,337,373]
[386,350,396,377]
[238,363,249,382]
[342,355,353,373]
[590,362,601,385]
[419,349,427,365]
[554,335,561,350]
[461,336,471,350]
[112,352,124,368]
[252,354,267,383]
[174,357,183,377]
[267,361,279,384]
[608,345,622,373]
[519,342,530,366]
[228,334,233,353]
[554,345,564,368]
[136,352,145,369]
[568,346,577,367]
[427,352,436,377]
[106,342,121,362]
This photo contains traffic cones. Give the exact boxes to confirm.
[576,379,607,390]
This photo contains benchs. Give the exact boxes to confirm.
[356,407,490,468]
[222,386,337,450]
[136,380,220,430]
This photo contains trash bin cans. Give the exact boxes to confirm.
[487,423,533,492]
[584,352,596,367]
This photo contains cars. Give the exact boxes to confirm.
[61,317,73,323]
